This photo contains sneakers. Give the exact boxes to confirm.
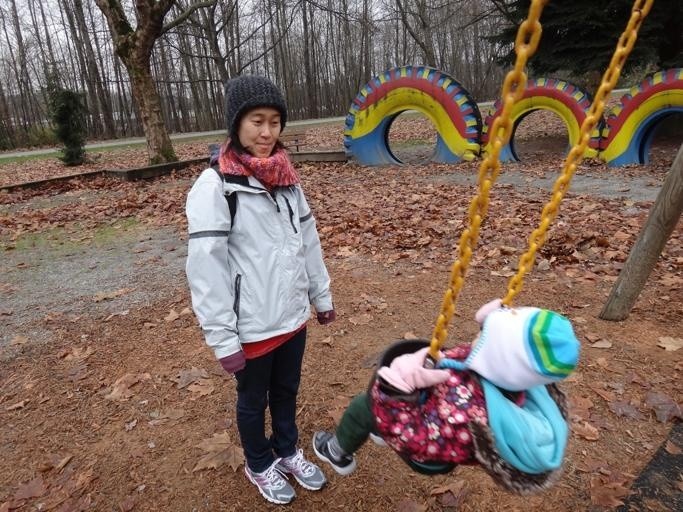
[271,447,327,494]
[311,430,357,477]
[367,430,390,449]
[242,457,297,506]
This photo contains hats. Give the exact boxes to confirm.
[464,304,581,394]
[221,73,287,138]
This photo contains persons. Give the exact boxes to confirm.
[182,73,335,508]
[308,291,584,498]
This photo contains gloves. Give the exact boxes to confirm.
[218,350,247,375]
[316,309,336,326]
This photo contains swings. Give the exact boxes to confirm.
[366,0,653,475]
